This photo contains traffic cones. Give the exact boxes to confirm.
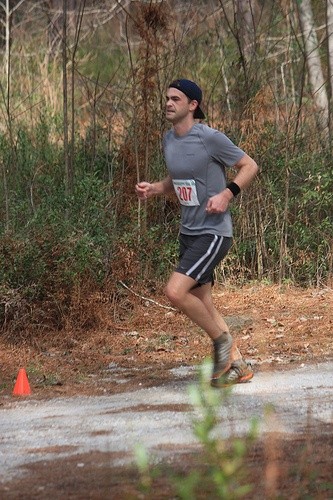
[13,366,32,395]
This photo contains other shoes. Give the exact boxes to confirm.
[210,361,254,387]
[210,334,236,380]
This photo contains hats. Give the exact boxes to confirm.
[168,79,206,120]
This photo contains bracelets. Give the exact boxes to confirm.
[226,183,241,197]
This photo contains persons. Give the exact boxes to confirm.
[136,80,258,388]
